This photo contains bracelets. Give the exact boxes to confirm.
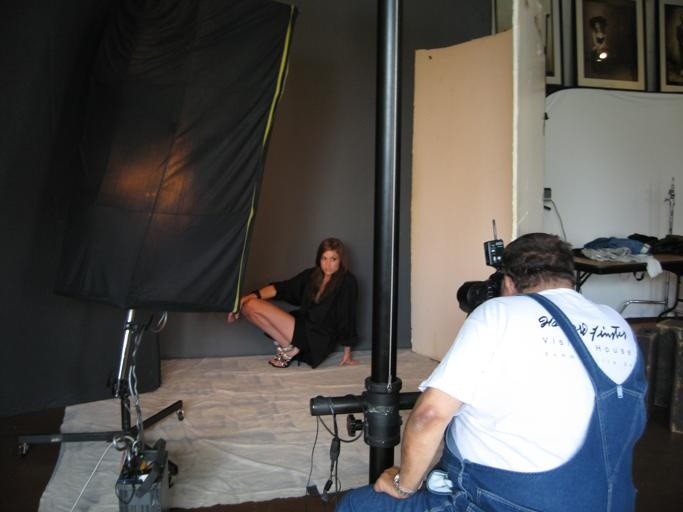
[252,290,262,300]
[393,469,425,497]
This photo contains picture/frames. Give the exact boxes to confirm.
[544,1,683,94]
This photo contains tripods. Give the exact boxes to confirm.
[19,310,183,490]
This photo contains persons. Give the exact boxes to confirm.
[227,237,362,368]
[331,234,652,512]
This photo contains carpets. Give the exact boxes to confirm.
[35,349,437,510]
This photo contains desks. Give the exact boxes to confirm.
[568,253,683,324]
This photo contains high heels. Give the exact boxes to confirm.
[268,343,301,368]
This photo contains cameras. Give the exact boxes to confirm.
[456,239,504,313]
